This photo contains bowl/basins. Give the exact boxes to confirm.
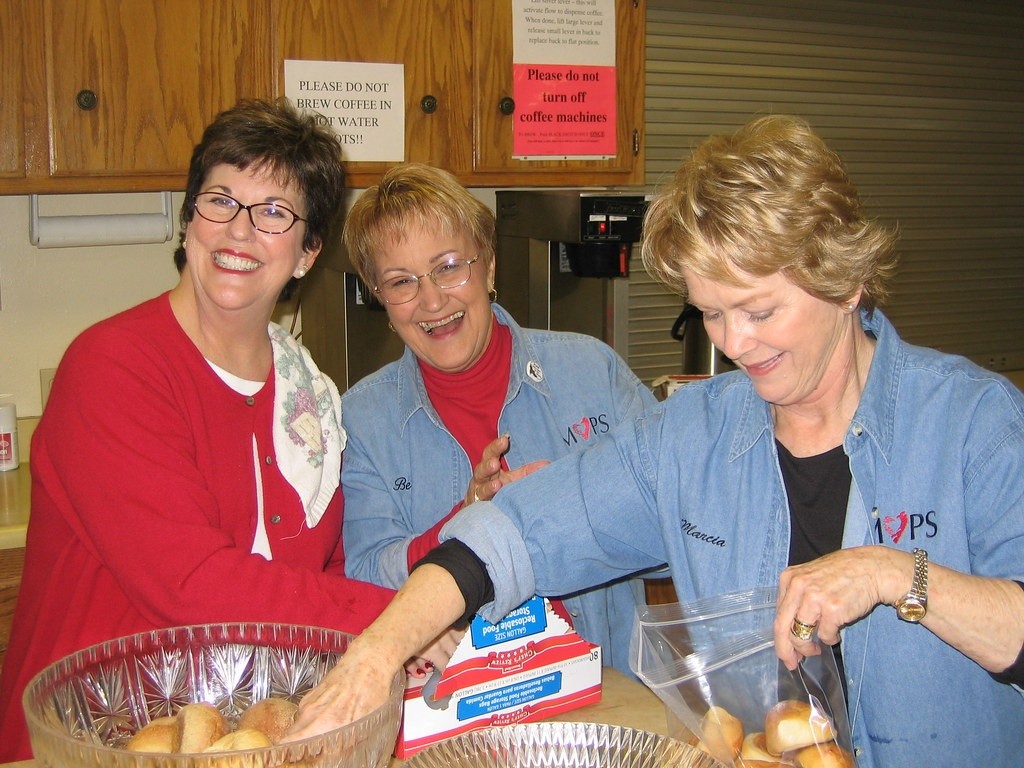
[22,622,406,768]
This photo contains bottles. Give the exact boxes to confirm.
[0,395,19,471]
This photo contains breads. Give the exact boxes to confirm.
[682,699,858,768]
[122,696,331,768]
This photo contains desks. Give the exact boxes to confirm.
[0,665,705,768]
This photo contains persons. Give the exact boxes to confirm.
[0,93,464,766]
[339,161,677,690]
[277,113,1024,768]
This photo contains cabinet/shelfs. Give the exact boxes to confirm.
[0,0,261,193]
[262,0,648,192]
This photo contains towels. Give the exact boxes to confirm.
[267,319,348,530]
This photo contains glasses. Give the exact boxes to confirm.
[374,254,479,305]
[191,192,309,234]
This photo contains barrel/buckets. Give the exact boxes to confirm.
[671,286,739,376]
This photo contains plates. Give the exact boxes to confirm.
[399,722,730,768]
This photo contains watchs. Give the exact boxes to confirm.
[894,548,928,622]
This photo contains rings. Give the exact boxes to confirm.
[475,486,481,503]
[790,618,816,640]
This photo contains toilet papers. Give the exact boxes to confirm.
[35,212,168,250]
